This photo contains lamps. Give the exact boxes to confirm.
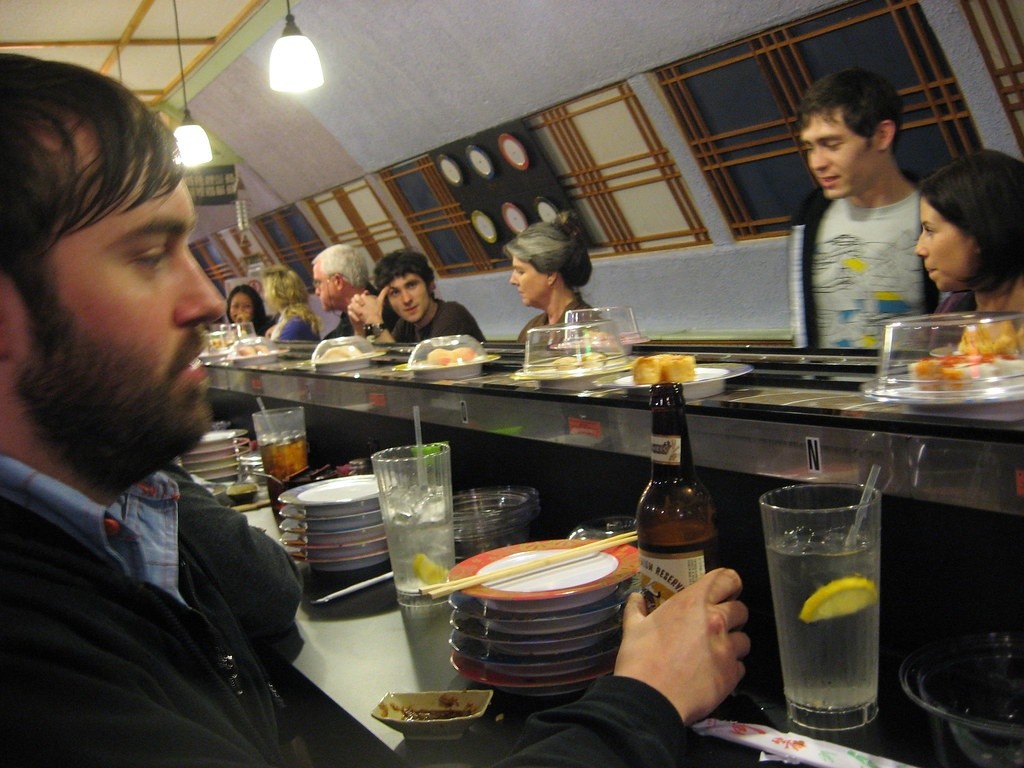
[171,0,214,166]
[267,0,323,93]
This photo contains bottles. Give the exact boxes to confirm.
[636,383,719,618]
[364,323,378,342]
[349,458,371,473]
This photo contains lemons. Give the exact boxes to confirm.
[800,577,878,623]
[413,554,448,586]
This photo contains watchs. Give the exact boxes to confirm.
[372,324,386,337]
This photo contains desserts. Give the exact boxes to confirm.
[427,347,474,366]
[552,352,606,370]
[907,352,1024,390]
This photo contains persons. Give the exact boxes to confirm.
[787,66,1024,358]
[0,52,749,768]
[226,242,487,345]
[503,211,603,344]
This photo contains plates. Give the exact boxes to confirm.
[448,538,640,695]
[438,133,563,244]
[276,474,397,572]
[509,356,640,390]
[198,350,289,367]
[929,346,957,356]
[591,363,753,399]
[452,485,540,542]
[175,429,251,485]
[392,353,502,381]
[872,359,1024,404]
[302,351,387,373]
[549,338,650,357]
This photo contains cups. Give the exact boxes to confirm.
[371,443,455,596]
[758,482,883,730]
[252,407,307,485]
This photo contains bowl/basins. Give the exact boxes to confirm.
[371,690,492,740]
[899,628,1024,768]
[226,485,256,504]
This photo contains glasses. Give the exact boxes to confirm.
[312,276,335,289]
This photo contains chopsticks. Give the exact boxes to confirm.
[419,531,638,600]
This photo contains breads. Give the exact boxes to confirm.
[631,354,696,385]
[239,345,269,356]
